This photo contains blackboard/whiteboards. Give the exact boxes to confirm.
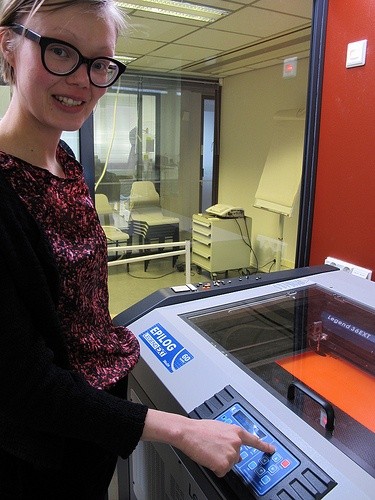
[252,124,305,217]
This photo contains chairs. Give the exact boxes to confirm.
[126,180,180,273]
[94,193,130,273]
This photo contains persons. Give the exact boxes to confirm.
[0,0,275,499]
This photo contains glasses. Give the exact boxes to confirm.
[10,22,128,88]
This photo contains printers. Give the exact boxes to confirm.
[205,203,244,219]
[108,263,375,500]
